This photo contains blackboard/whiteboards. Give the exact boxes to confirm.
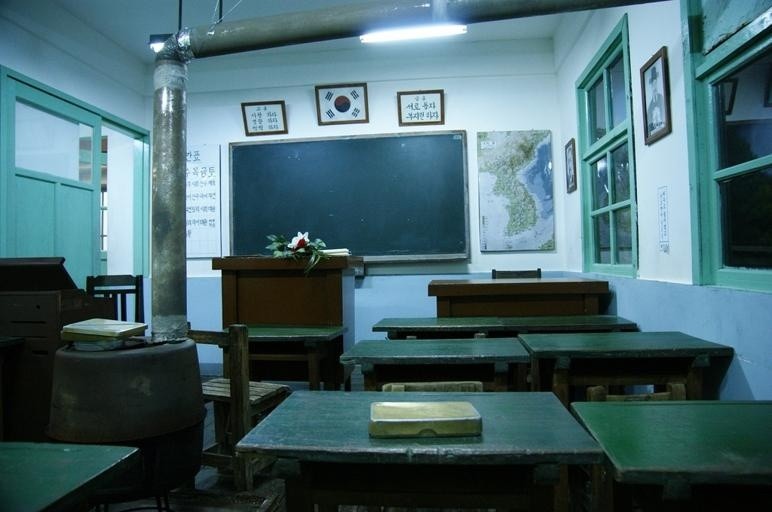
[229,130,470,261]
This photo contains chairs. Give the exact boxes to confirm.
[87,274,146,324]
[493,267,543,280]
[186,321,292,494]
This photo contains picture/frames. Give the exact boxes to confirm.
[563,137,581,194]
[639,47,674,146]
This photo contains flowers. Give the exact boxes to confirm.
[264,228,329,270]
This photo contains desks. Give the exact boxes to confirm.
[1,439,147,512]
